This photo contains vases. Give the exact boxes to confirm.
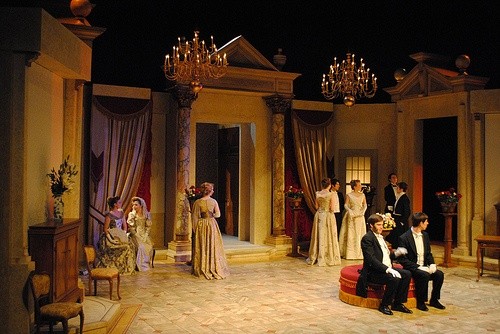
[288,198,301,209]
[189,200,195,212]
[441,202,456,212]
[54,196,65,222]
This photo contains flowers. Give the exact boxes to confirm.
[436,188,462,204]
[47,154,78,197]
[377,212,396,229]
[286,184,304,199]
[184,185,203,202]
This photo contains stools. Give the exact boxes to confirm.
[340,266,417,308]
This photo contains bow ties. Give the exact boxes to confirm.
[416,234,423,238]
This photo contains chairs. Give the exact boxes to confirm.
[29,270,84,334]
[83,245,121,303]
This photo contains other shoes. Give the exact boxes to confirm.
[429,299,445,310]
[417,302,429,311]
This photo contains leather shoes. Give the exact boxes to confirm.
[391,302,413,314]
[378,304,393,315]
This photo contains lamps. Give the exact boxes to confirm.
[321,51,378,109]
[164,31,227,98]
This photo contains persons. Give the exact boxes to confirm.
[329,178,345,242]
[338,180,367,260]
[191,182,230,279]
[95,196,136,276]
[392,212,445,311]
[305,178,341,266]
[384,173,411,227]
[356,214,413,315]
[126,197,155,272]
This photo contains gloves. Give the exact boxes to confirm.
[430,264,437,273]
[394,247,408,257]
[417,266,432,274]
[386,267,402,278]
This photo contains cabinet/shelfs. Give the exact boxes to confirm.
[28,216,84,308]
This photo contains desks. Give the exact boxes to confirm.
[474,235,500,281]
